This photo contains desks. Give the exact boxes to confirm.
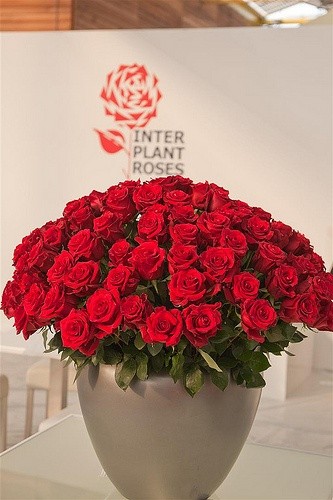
[1,413,331,500]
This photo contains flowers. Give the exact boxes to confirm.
[0,175,332,401]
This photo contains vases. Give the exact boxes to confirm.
[70,356,262,500]
